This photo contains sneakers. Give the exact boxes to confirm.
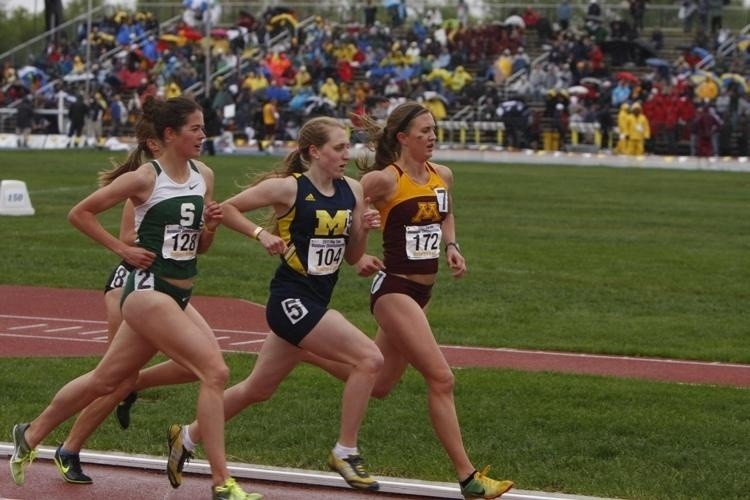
[115,391,137,429]
[54,443,93,484]
[327,450,379,490]
[167,424,193,488]
[460,472,514,500]
[9,423,38,485]
[212,478,264,500]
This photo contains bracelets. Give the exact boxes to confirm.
[203,223,218,234]
[445,242,461,252]
[252,227,264,240]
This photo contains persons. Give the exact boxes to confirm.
[300,103,514,500]
[11,96,265,500]
[2,1,750,156]
[54,95,225,484]
[168,116,385,491]
